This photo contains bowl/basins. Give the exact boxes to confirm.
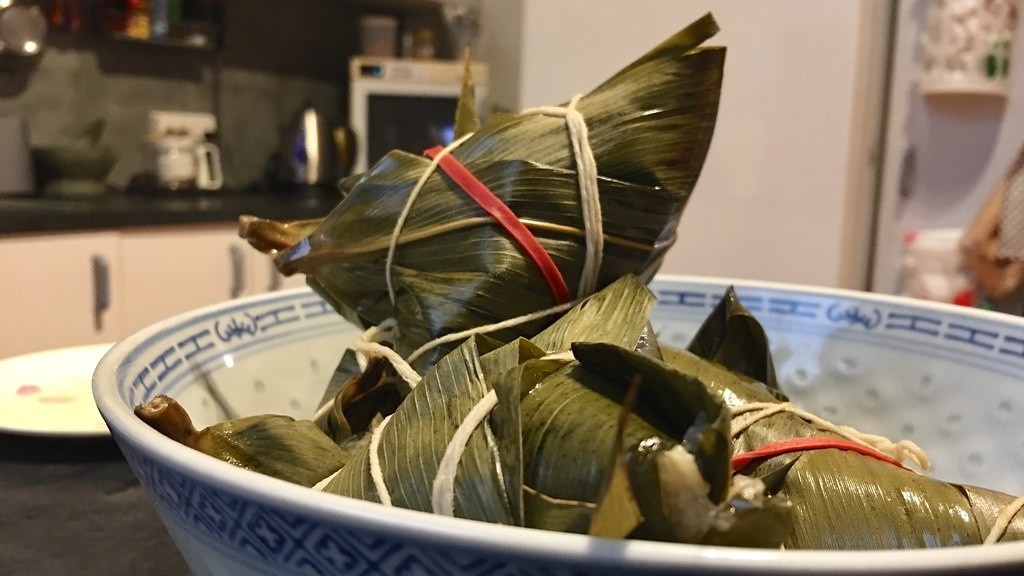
[95,276,1024,576]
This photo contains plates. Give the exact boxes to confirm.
[0,344,114,438]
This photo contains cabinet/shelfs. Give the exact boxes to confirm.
[1,231,121,362]
[121,225,309,343]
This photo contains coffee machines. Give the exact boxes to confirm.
[144,112,223,194]
